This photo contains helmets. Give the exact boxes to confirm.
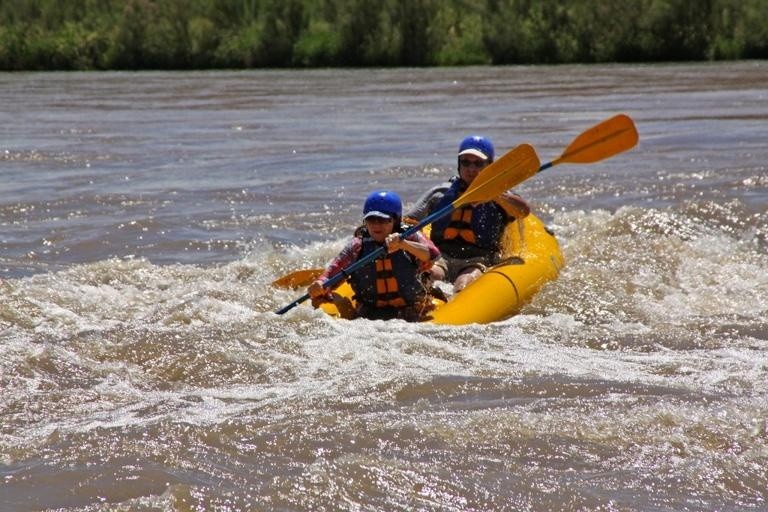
[456,134,496,161]
[361,190,403,219]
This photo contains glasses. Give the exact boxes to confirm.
[364,217,392,224]
[460,159,485,167]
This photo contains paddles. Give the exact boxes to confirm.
[271,114,639,288]
[275,144,540,314]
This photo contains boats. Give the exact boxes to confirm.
[319,212,564,326]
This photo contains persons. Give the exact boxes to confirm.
[307,185,432,328]
[401,133,512,298]
